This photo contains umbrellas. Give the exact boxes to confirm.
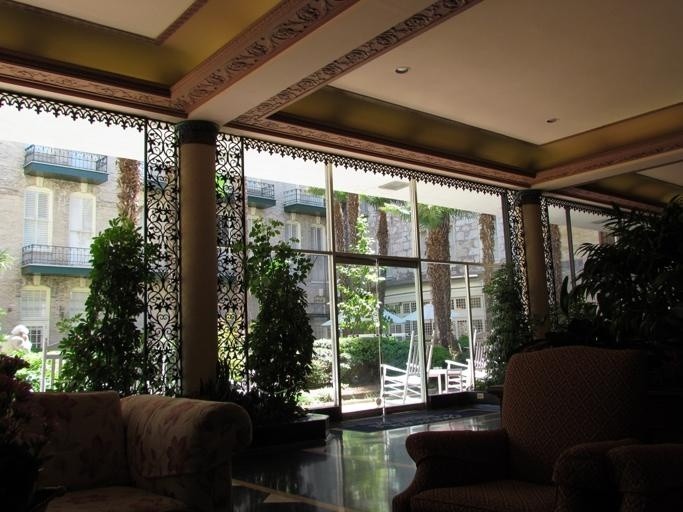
[403,302,460,330]
[321,309,406,333]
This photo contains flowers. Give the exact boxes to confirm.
[0,353,55,479]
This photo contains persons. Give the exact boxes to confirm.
[2,324,32,353]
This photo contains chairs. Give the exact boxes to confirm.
[379,329,436,405]
[390,336,683,511]
[15,387,254,512]
[444,329,501,392]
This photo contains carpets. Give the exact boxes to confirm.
[333,406,498,433]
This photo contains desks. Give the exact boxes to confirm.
[426,367,463,395]
